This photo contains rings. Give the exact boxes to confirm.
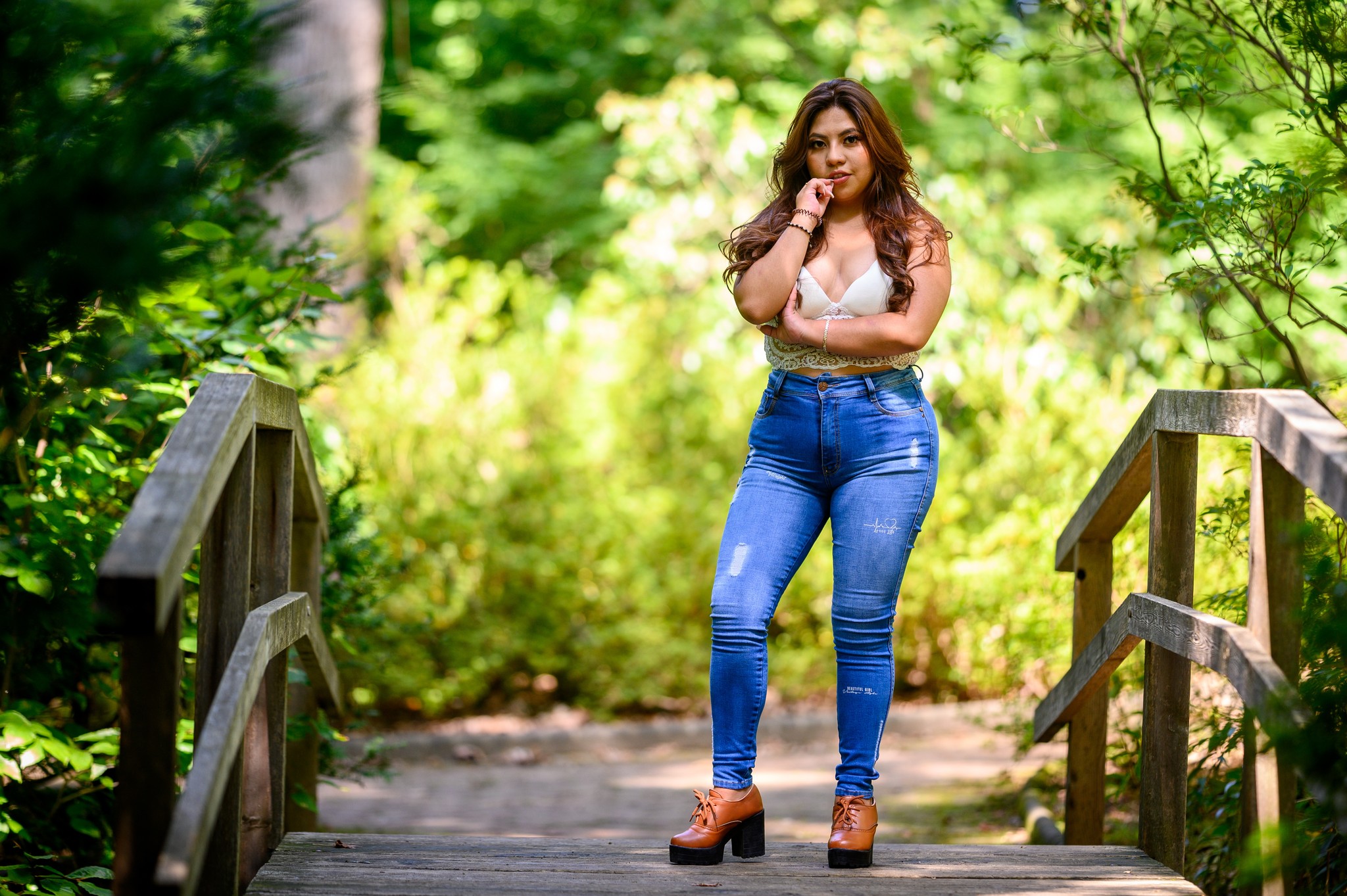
[805,183,808,185]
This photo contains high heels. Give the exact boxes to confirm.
[669,784,765,866]
[827,795,878,869]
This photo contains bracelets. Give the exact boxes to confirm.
[787,222,813,248]
[823,320,830,356]
[792,208,822,228]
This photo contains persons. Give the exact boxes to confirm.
[667,78,954,872]
